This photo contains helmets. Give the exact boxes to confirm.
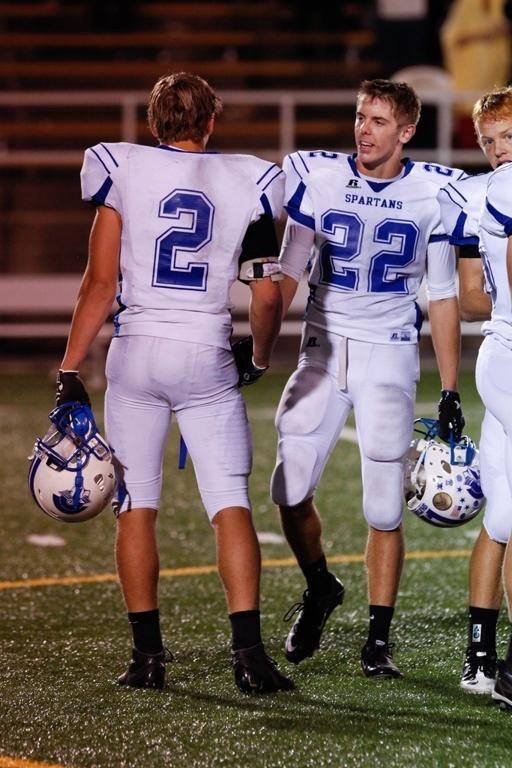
[406,437,485,527]
[28,401,118,523]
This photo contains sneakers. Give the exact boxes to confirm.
[119,650,167,689]
[286,578,345,665]
[461,645,512,707]
[361,641,401,679]
[233,656,295,693]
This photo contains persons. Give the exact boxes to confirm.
[52,73,298,694]
[474,92,512,707]
[261,78,466,683]
[438,89,511,696]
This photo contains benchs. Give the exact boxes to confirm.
[1,1,512,174]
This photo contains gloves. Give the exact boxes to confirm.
[53,369,89,409]
[436,390,464,440]
[231,337,267,387]
